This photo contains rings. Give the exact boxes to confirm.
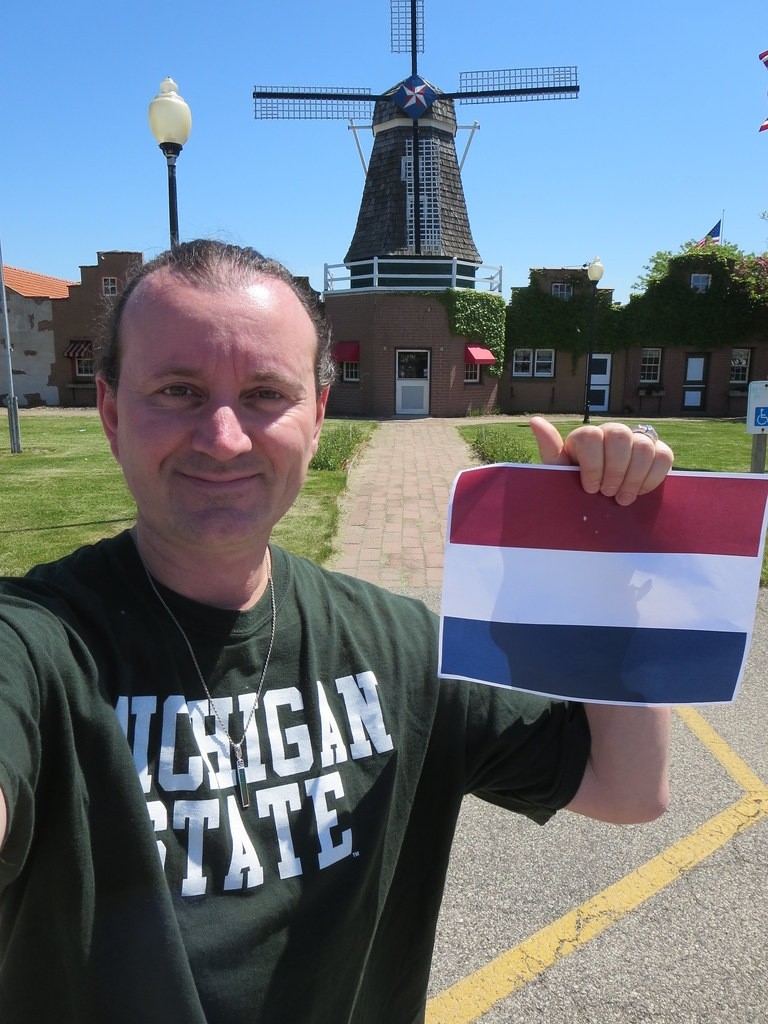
[632,424,658,443]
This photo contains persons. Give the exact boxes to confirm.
[0,238,675,1024]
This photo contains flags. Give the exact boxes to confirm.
[439,461,768,708]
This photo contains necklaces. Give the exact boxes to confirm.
[142,554,279,810]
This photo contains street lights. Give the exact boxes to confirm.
[148,76,192,246]
[583,255,604,424]
